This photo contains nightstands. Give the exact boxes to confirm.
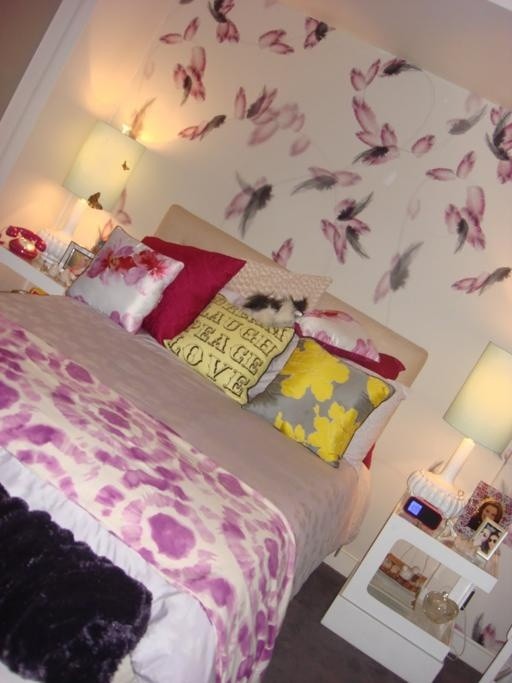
[321,498,498,683]
[0,228,69,296]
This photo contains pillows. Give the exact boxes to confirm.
[295,309,379,365]
[220,257,332,402]
[66,226,184,334]
[241,338,396,469]
[163,293,296,407]
[293,322,406,470]
[141,235,246,345]
[247,333,407,474]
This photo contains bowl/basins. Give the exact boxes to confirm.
[423,591,459,624]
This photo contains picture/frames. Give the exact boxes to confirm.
[468,516,508,561]
[59,241,95,279]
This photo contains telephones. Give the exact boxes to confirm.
[5,224,48,262]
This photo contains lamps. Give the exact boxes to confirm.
[407,340,512,519]
[35,120,146,267]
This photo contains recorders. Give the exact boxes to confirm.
[403,494,444,532]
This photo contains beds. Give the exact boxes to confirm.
[0,204,429,683]
